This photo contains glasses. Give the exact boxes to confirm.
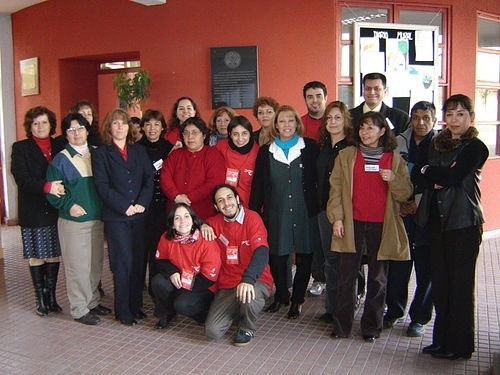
[66,126,85,135]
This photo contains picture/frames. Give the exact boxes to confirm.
[20,56,40,98]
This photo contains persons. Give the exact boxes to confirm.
[148,202,222,329]
[44,101,112,323]
[299,81,328,294]
[410,94,489,360]
[327,111,413,342]
[11,106,65,316]
[382,101,439,338]
[208,107,238,148]
[317,101,356,323]
[346,72,410,312]
[137,109,180,292]
[251,97,297,263]
[249,105,326,320]
[161,97,201,146]
[214,115,264,212]
[159,117,227,227]
[194,184,274,345]
[91,109,154,326]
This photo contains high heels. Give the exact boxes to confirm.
[431,348,472,360]
[266,296,289,312]
[421,343,442,353]
[288,301,302,319]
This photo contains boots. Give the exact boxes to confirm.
[44,260,63,312]
[28,262,49,315]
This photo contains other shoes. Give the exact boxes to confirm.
[383,311,399,327]
[193,312,206,325]
[407,320,425,336]
[364,334,376,342]
[154,310,176,328]
[330,332,348,340]
[97,281,104,296]
[115,312,138,326]
[131,308,148,320]
[319,312,333,323]
[355,288,366,308]
[384,304,389,313]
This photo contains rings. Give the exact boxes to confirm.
[385,176,386,178]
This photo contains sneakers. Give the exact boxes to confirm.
[307,281,327,296]
[234,327,255,347]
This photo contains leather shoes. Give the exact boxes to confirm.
[75,312,98,325]
[89,304,112,316]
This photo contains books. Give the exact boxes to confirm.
[168,141,182,156]
